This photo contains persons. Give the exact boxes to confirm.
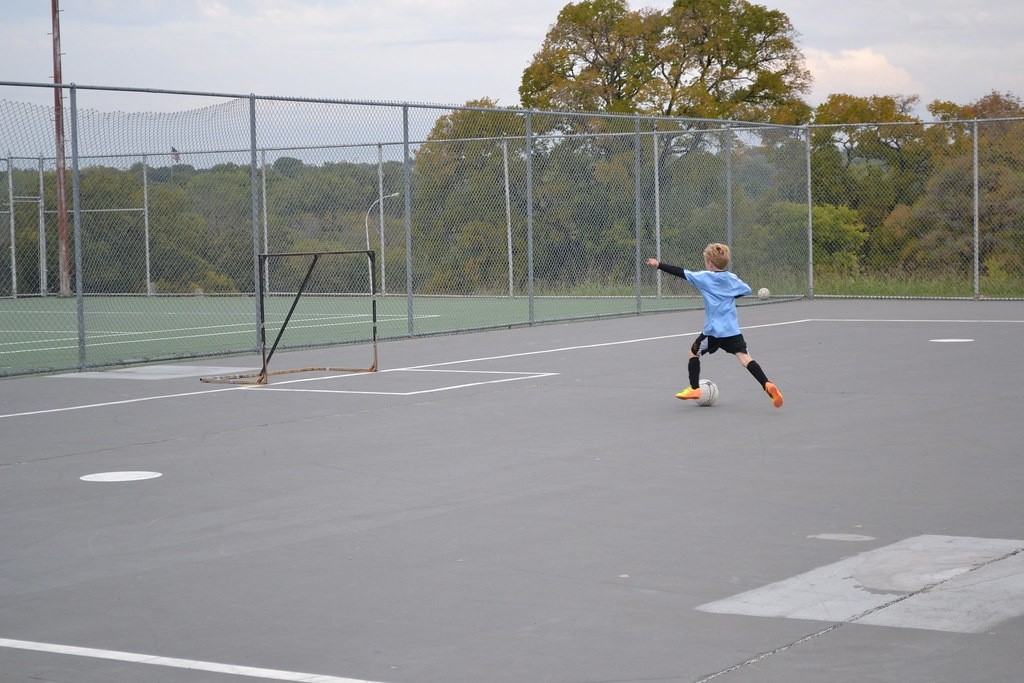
[647,243,783,408]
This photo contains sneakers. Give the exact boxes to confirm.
[766,382,783,407]
[676,386,700,400]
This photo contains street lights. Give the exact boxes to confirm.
[365,192,399,299]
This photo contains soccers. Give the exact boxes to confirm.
[694,379,720,407]
[758,288,770,300]
